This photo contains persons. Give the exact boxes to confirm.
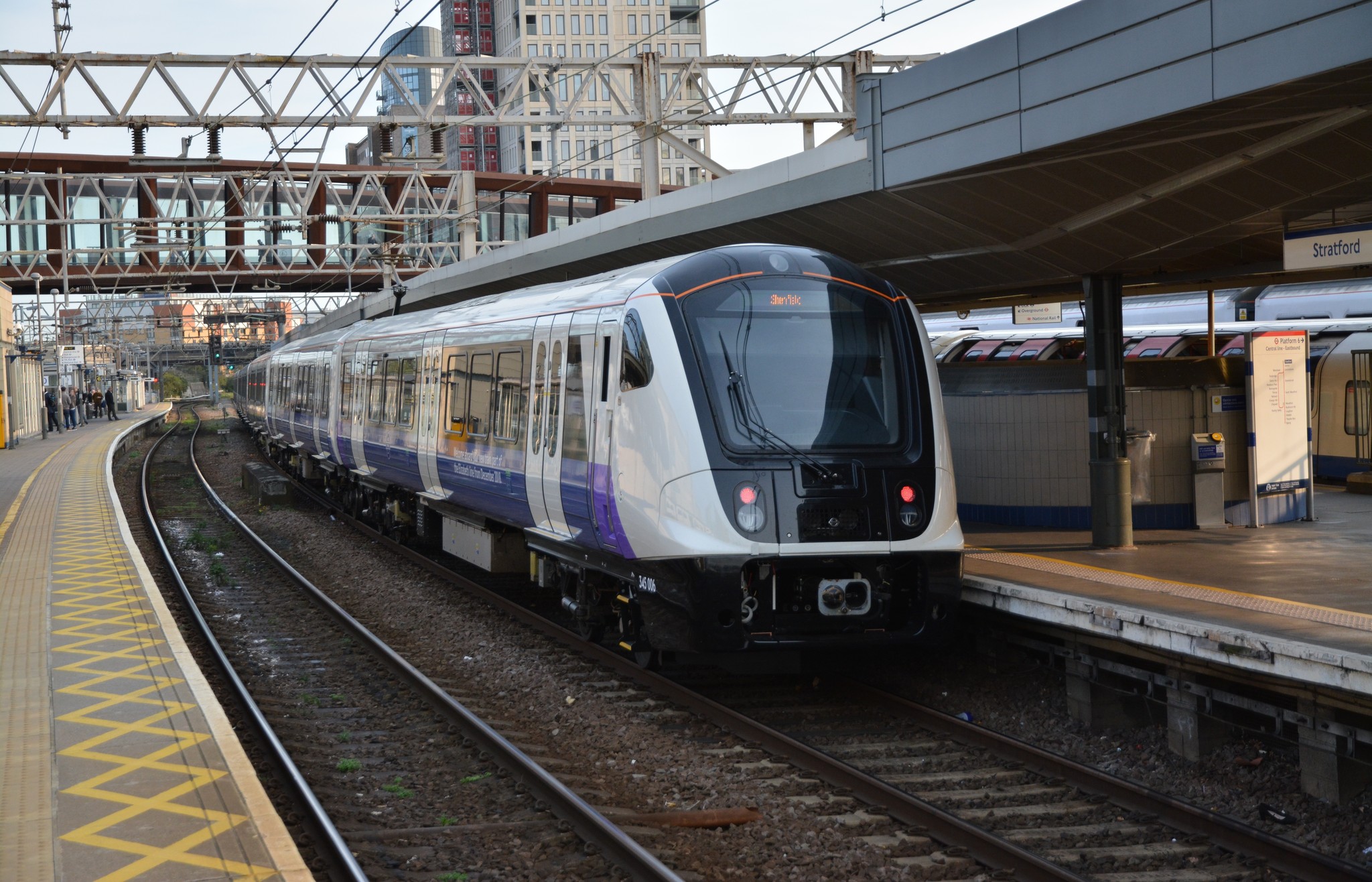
[44,388,59,432]
[68,389,77,431]
[72,386,89,425]
[105,387,121,421]
[61,386,73,431]
[87,387,104,418]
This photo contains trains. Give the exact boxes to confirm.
[232,241,967,671]
[919,277,1372,484]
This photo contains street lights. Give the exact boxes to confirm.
[30,271,51,441]
[54,323,149,416]
[51,287,68,434]
[14,305,44,350]
[82,294,91,345]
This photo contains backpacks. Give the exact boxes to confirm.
[45,392,58,407]
[101,397,106,408]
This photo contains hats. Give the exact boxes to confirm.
[73,387,76,389]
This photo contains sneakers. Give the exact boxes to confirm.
[72,427,77,430]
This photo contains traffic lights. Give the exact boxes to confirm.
[213,347,223,365]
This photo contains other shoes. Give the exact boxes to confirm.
[56,429,59,431]
[77,422,81,425]
[100,416,102,418]
[67,428,72,431]
[49,429,53,432]
[115,418,121,420]
[109,419,113,421]
[85,421,89,425]
